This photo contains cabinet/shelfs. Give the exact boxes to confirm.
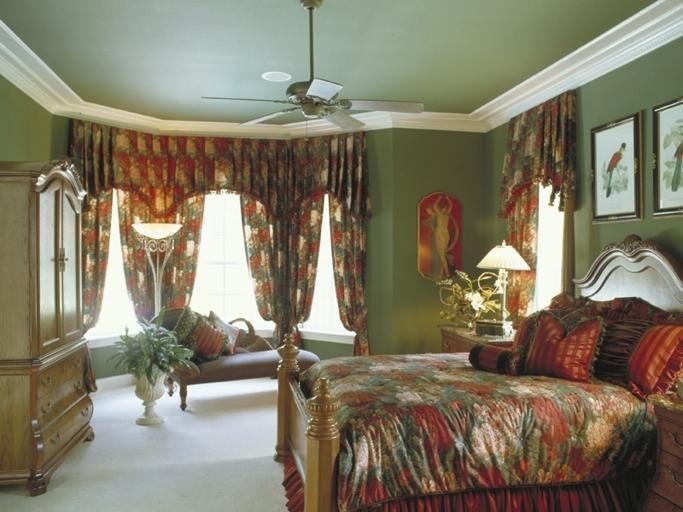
[0,158,95,498]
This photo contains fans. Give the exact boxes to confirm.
[200,0,423,132]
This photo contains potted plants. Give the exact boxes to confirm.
[105,309,194,426]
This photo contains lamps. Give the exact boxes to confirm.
[476,241,530,338]
[131,223,182,315]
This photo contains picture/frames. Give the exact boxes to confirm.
[652,97,682,216]
[590,109,644,225]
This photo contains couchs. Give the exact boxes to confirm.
[151,308,318,411]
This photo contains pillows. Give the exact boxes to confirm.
[600,321,677,395]
[521,310,606,383]
[467,342,522,377]
[513,310,599,382]
[467,343,517,372]
[207,314,242,355]
[187,315,222,359]
[624,323,682,400]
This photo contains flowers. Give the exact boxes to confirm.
[461,285,494,314]
[273,235,681,512]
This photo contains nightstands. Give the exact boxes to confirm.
[642,399,683,512]
